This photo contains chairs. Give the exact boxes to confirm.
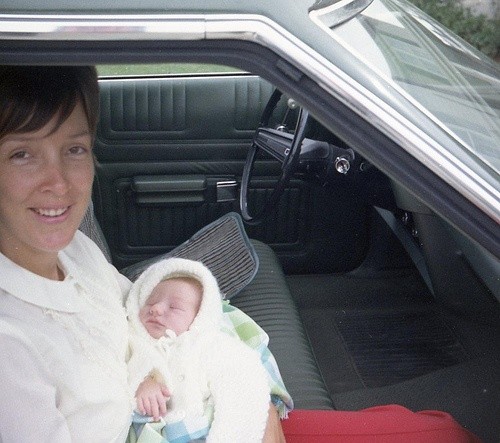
[77,196,337,414]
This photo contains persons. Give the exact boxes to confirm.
[125,258,271,443]
[0,64,487,443]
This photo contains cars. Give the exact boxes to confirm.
[1,0,500,443]
